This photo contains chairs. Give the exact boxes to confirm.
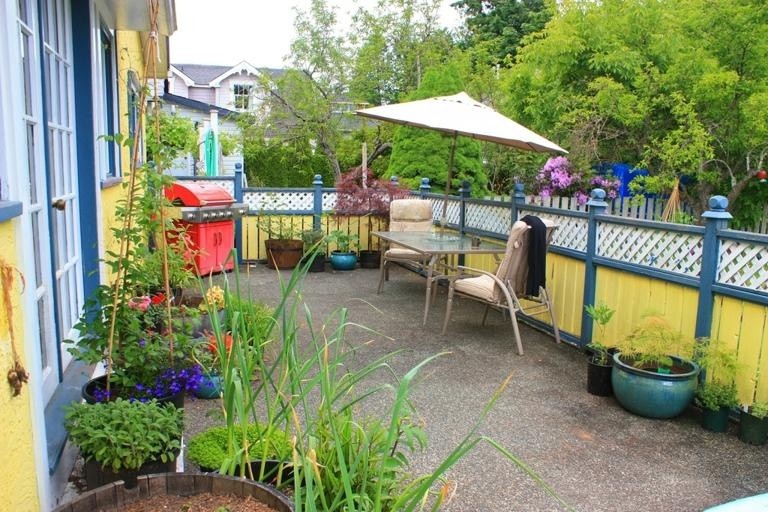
[376,198,565,355]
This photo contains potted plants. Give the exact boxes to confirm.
[187,238,329,511]
[583,302,767,449]
[61,398,182,491]
[330,155,410,269]
[255,192,330,273]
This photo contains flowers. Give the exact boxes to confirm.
[133,361,206,401]
[127,292,165,311]
[93,388,110,403]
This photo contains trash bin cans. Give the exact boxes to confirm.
[151,182,238,277]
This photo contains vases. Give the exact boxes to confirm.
[81,368,183,410]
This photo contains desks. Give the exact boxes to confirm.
[369,230,510,327]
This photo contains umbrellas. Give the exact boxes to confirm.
[350,89,570,238]
